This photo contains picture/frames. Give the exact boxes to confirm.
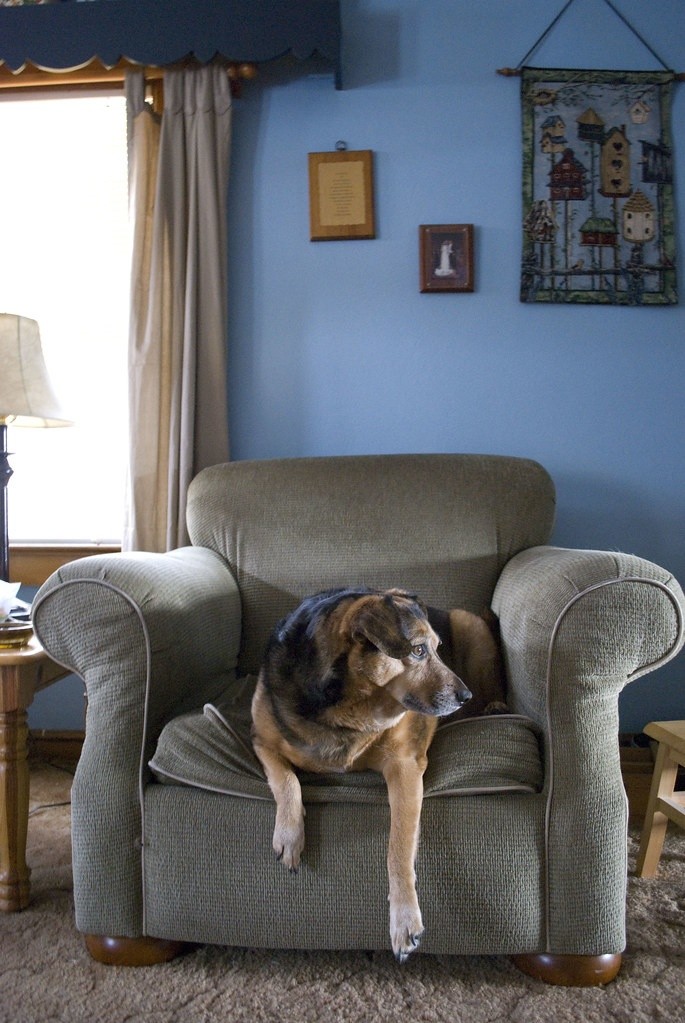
[417,224,472,292]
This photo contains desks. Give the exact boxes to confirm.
[1,637,76,916]
[30,452,685,989]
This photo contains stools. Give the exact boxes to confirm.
[638,719,685,881]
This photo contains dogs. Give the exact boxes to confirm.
[249,584,511,964]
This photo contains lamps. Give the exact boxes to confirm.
[0,312,71,646]
